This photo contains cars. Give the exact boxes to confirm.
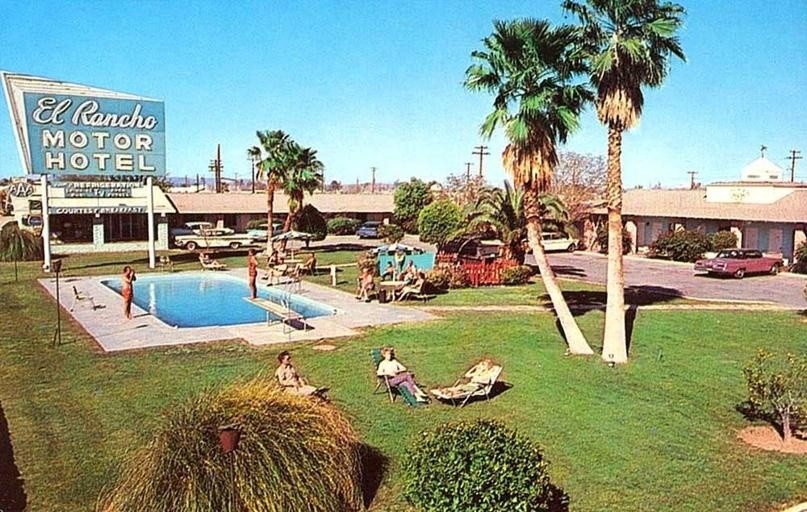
[356,218,387,242]
[521,232,581,257]
[692,247,783,278]
[169,214,288,250]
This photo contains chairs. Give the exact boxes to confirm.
[369,348,433,410]
[274,368,330,406]
[69,285,96,313]
[196,255,228,272]
[266,255,318,294]
[429,359,505,409]
[353,264,432,304]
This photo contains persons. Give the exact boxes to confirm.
[275,350,329,405]
[268,250,283,272]
[248,248,259,300]
[439,359,493,396]
[291,252,315,278]
[200,252,221,266]
[122,266,136,319]
[376,346,430,402]
[355,267,374,302]
[376,259,425,301]
